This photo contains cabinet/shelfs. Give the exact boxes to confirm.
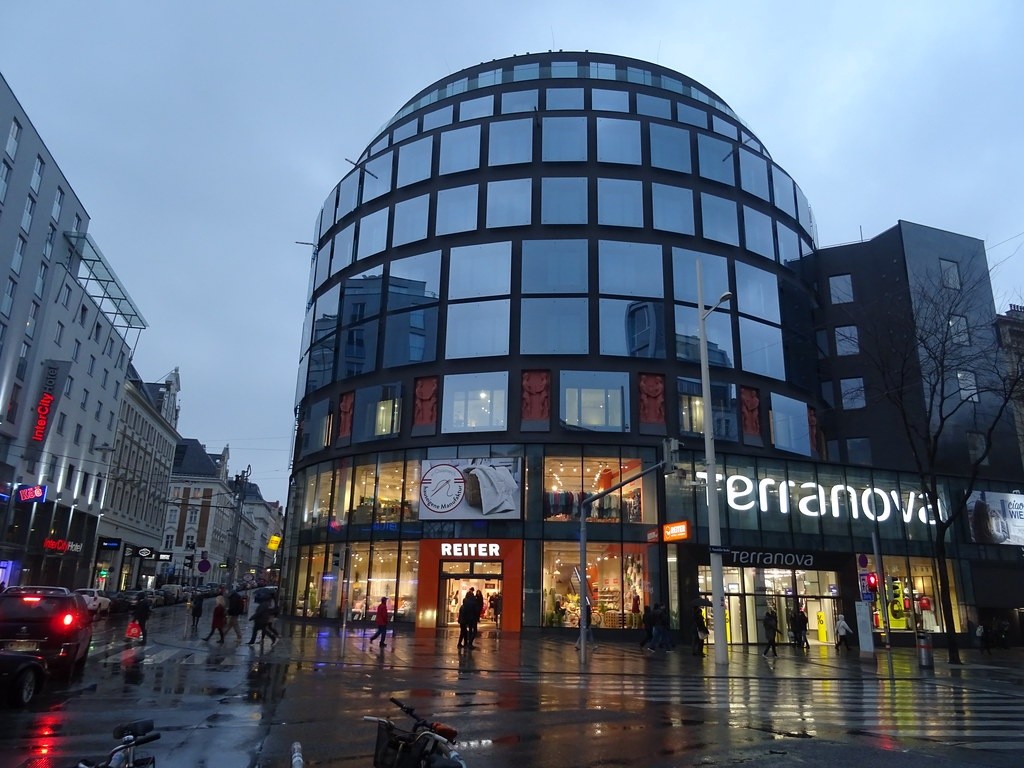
[351,496,414,523]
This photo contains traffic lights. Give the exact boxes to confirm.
[867,574,878,593]
[183,553,194,569]
[661,437,685,475]
[187,542,195,549]
[886,575,900,602]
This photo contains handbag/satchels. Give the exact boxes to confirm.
[836,629,838,635]
[125,621,142,640]
[698,630,707,639]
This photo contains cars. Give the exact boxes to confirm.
[104,578,270,614]
[0,648,48,710]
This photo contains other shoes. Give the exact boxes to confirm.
[271,637,276,646]
[216,640,224,643]
[575,645,581,650]
[245,642,254,645]
[647,647,655,652]
[457,643,463,647]
[201,638,208,642]
[469,645,476,650]
[762,654,768,658]
[370,638,372,644]
[380,643,387,647]
[593,646,598,650]
[256,641,264,644]
[774,656,780,658]
[666,650,675,653]
[835,647,840,651]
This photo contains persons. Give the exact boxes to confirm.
[245,572,282,646]
[545,587,566,627]
[975,615,1009,655]
[191,588,203,629]
[639,603,675,653]
[574,605,599,650]
[370,597,389,647]
[632,589,640,629]
[788,605,810,649]
[835,615,853,651]
[449,588,497,650]
[762,612,779,659]
[200,596,226,644]
[224,584,244,642]
[0,581,6,593]
[124,591,151,644]
[692,607,709,658]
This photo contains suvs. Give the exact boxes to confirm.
[0,585,92,686]
[73,588,112,621]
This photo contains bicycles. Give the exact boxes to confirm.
[75,718,161,768]
[375,696,467,768]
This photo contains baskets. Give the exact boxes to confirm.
[375,719,429,768]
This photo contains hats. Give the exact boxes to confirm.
[381,597,388,601]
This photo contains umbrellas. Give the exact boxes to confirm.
[763,621,783,635]
[687,599,713,609]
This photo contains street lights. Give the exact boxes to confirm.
[695,257,736,665]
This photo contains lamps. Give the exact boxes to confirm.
[57,492,62,500]
[99,509,105,516]
[89,504,94,511]
[73,498,79,506]
